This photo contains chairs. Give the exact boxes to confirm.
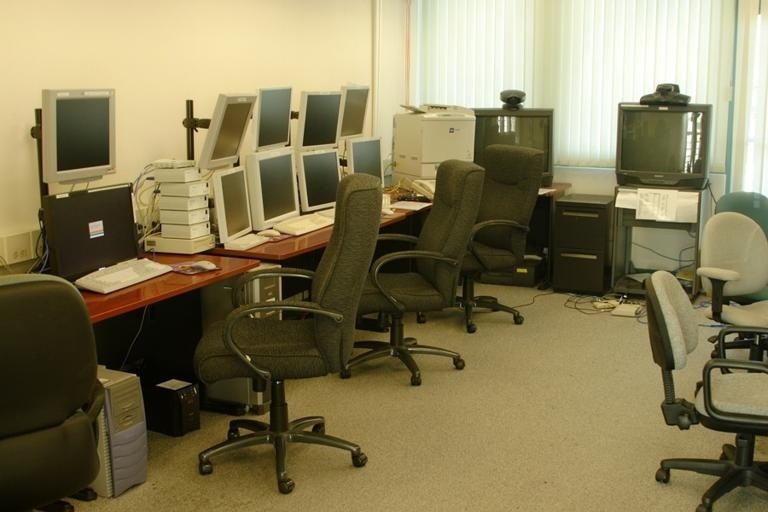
[696,210,768,359]
[196,174,382,492]
[642,270,768,512]
[0,274,105,512]
[715,192,768,343]
[340,159,486,385]
[417,144,543,334]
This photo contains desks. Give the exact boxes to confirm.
[69,248,259,323]
[214,202,431,301]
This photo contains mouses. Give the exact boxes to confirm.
[258,229,279,237]
[192,259,218,271]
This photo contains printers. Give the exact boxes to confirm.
[392,104,476,192]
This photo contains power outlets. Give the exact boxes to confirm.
[135,206,161,234]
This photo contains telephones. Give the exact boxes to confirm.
[398,180,435,203]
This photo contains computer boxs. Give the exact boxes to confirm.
[94,366,146,497]
[156,377,200,437]
[199,263,283,414]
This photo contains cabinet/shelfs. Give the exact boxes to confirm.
[553,194,615,297]
[611,179,713,306]
[526,184,572,295]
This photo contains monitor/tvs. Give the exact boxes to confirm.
[257,87,292,151]
[43,186,143,284]
[343,87,372,140]
[243,150,301,231]
[212,166,252,247]
[472,109,554,187]
[345,140,386,192]
[296,91,346,152]
[198,93,258,171]
[40,88,116,183]
[616,102,712,189]
[301,148,346,213]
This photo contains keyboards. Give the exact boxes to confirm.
[224,235,269,251]
[272,215,335,238]
[73,258,174,294]
